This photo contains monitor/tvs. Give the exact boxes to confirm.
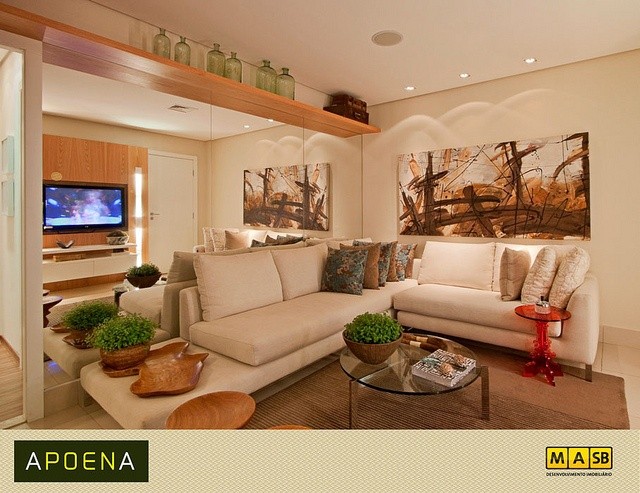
[42,180,129,232]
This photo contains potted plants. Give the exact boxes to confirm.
[104,230,129,245]
[343,311,406,365]
[123,263,161,289]
[87,313,158,376]
[57,299,121,349]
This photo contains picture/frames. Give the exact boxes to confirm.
[1,136,14,179]
[1,178,14,217]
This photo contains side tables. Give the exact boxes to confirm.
[516,303,572,386]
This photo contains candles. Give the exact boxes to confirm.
[540,296,544,302]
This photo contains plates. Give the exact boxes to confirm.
[164,391,256,430]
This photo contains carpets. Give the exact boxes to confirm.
[238,344,631,429]
[45,296,118,327]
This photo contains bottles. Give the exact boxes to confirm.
[174,35,191,67]
[275,68,295,100]
[535,295,551,314]
[206,43,225,77]
[152,28,171,59]
[224,51,242,83]
[256,59,277,96]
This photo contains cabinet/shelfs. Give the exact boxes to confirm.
[42,243,140,291]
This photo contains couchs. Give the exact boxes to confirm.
[42,245,331,380]
[80,239,420,430]
[393,239,599,383]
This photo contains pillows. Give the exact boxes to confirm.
[286,234,303,244]
[492,242,576,292]
[500,248,532,301]
[166,252,199,283]
[321,246,368,296]
[277,234,295,245]
[202,228,212,251]
[164,247,249,285]
[265,235,277,244]
[396,242,412,281]
[339,242,381,290]
[247,228,267,247]
[521,245,559,305]
[352,240,393,287]
[224,230,254,253]
[268,231,302,240]
[550,247,591,311]
[191,250,284,322]
[417,240,495,292]
[272,243,331,300]
[250,240,267,246]
[405,242,418,279]
[211,228,238,252]
[387,241,399,282]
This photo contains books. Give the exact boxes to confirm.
[412,349,477,389]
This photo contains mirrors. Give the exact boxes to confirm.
[43,44,362,425]
